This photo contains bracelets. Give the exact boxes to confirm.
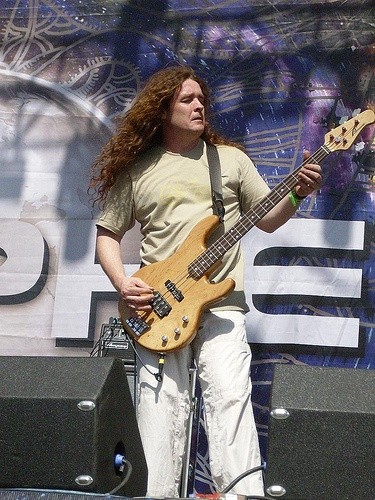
[288,192,303,208]
[291,187,307,200]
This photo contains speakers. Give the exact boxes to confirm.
[264,364,374,500]
[0,356,147,496]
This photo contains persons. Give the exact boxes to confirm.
[89,61,326,498]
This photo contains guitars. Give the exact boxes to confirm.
[118,108,375,354]
